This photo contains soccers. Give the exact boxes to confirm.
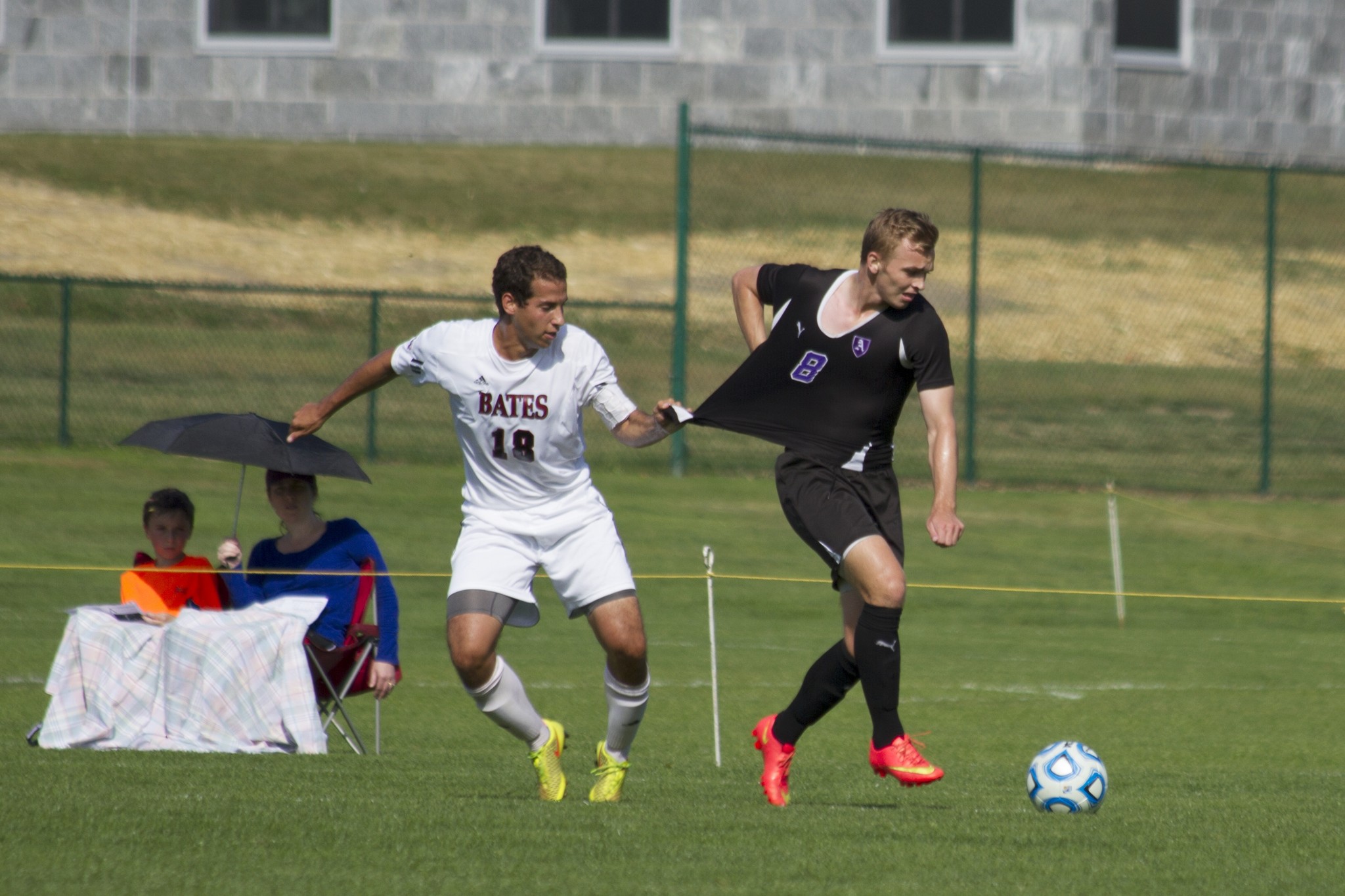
[1027,740,1108,813]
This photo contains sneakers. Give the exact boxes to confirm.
[869,733,944,788]
[752,714,797,807]
[590,740,632,805]
[528,719,570,802]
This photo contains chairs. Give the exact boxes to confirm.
[303,555,402,754]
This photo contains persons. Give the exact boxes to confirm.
[120,487,233,620]
[287,244,693,802]
[215,468,399,699]
[658,210,963,808]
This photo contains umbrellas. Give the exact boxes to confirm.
[113,410,373,563]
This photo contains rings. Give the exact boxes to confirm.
[388,682,394,686]
